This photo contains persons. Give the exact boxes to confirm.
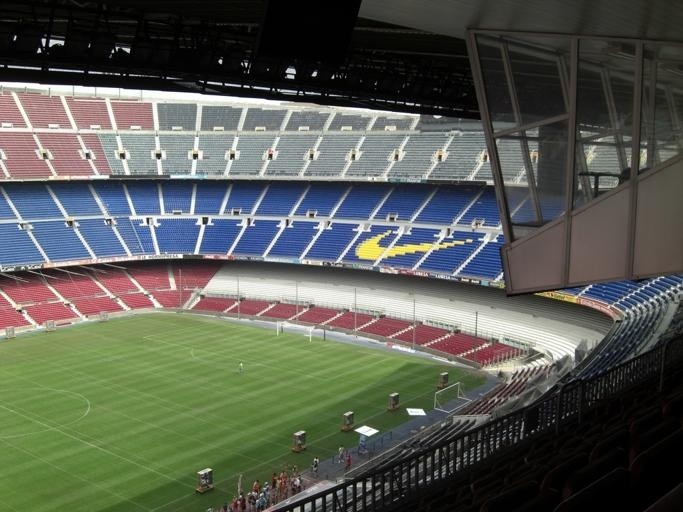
[207,440,367,512]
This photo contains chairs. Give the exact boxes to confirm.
[343,417,458,510]
[1,90,681,327]
[495,328,683,511]
[352,325,494,366]
[458,396,495,511]
